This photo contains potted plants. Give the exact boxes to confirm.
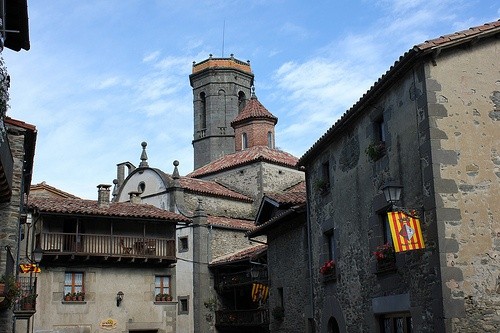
[156,294,171,301]
[65,292,84,301]
[0,273,36,310]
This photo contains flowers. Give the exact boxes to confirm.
[320,260,338,277]
[372,242,394,265]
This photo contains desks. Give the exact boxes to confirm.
[134,242,148,255]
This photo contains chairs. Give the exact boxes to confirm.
[120,239,133,254]
[147,241,156,255]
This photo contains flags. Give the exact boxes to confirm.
[18,263,41,274]
[387,209,426,253]
[252,283,269,304]
